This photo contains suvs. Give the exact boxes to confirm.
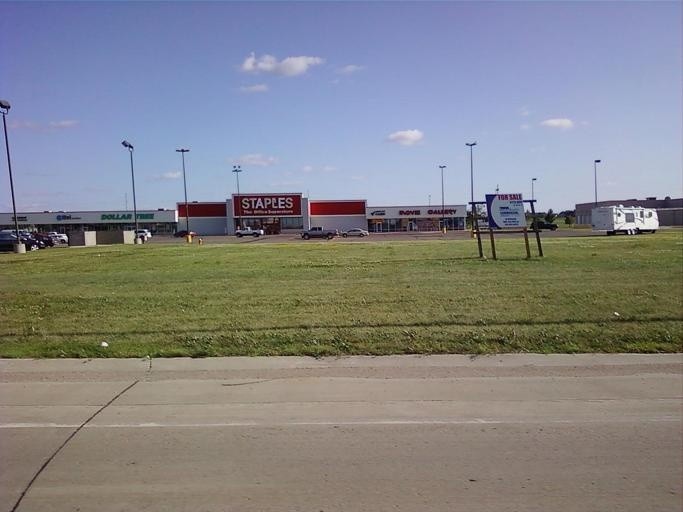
[531,221,558,231]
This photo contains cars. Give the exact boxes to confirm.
[0,229,69,251]
[136,229,151,238]
[173,230,196,237]
[341,227,369,237]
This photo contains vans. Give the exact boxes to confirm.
[592,206,659,235]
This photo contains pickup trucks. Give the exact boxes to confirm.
[235,227,264,237]
[301,226,339,239]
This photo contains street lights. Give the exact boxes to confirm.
[595,160,600,207]
[0,100,21,245]
[122,141,139,239]
[175,149,191,241]
[439,165,446,233]
[532,179,537,200]
[232,165,243,229]
[465,143,476,237]
